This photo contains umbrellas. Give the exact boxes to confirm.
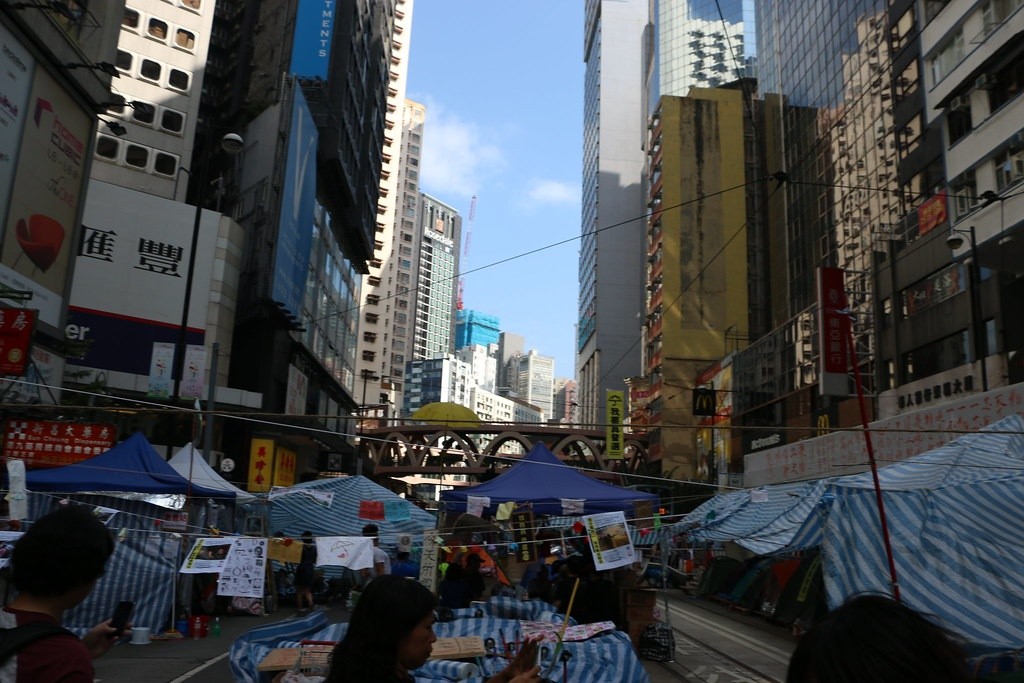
[408,402,481,440]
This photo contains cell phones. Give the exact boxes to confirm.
[523,645,539,674]
[107,600,135,639]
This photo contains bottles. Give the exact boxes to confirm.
[214,617,221,637]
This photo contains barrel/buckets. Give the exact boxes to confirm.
[175,620,187,635]
[190,615,208,638]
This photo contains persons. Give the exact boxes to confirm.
[319,574,542,683]
[360,524,391,583]
[292,532,318,613]
[785,595,993,683]
[1,506,134,683]
[448,553,485,607]
[644,532,691,589]
[576,566,621,626]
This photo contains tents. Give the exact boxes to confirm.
[7,432,258,503]
[438,441,659,515]
[550,413,1024,657]
[267,474,437,594]
